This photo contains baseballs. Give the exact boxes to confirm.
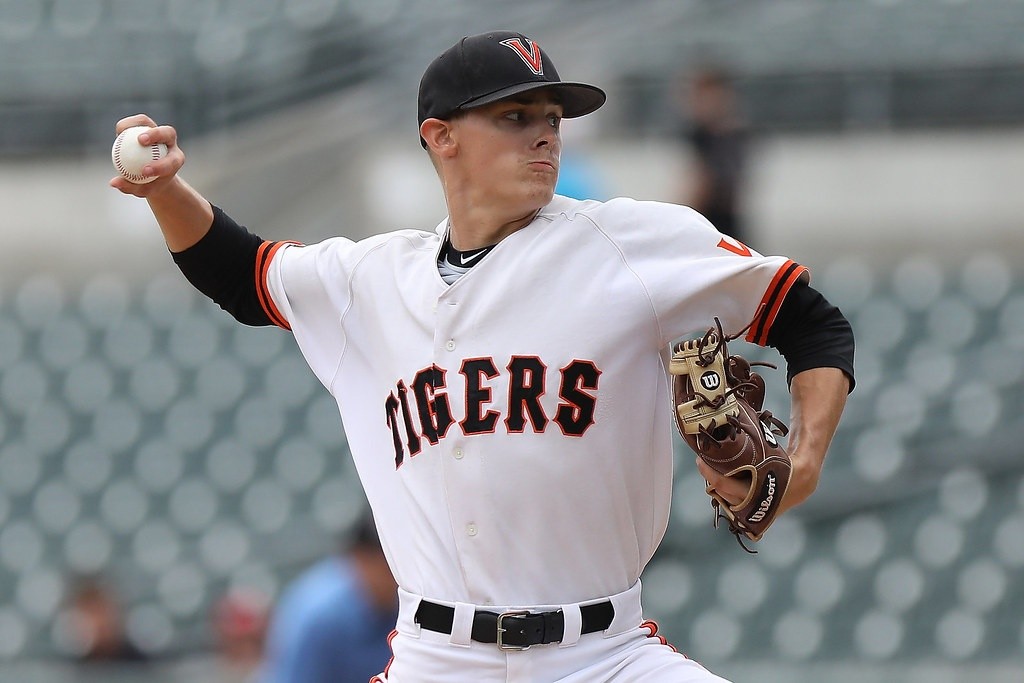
[113,124,168,184]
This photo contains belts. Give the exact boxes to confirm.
[414,598,614,651]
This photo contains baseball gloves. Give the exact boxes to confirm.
[666,317,793,559]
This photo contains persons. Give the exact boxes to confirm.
[253,495,398,680]
[662,43,758,245]
[109,30,856,683]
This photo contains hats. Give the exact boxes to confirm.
[417,31,608,149]
